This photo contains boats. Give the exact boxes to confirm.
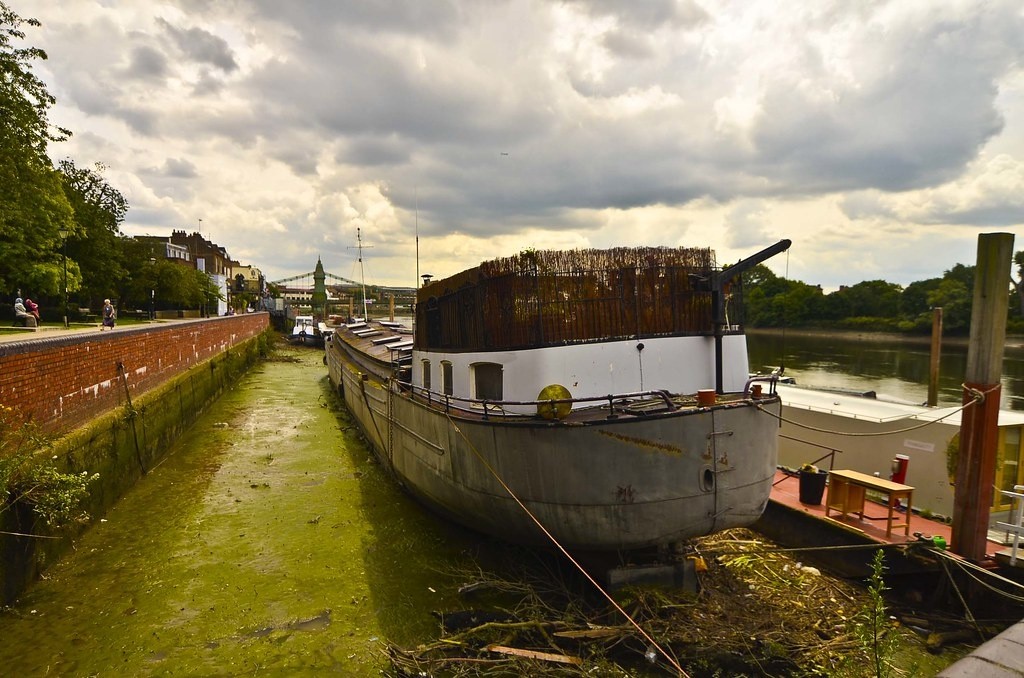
[289,187,792,555]
[751,374,1024,579]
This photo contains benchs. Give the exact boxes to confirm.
[78,308,98,323]
[12,316,27,327]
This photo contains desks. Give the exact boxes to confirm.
[825,470,915,537]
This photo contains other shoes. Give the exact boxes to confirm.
[100,329,104,331]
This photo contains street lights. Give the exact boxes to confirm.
[56,224,70,327]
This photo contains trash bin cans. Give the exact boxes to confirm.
[798,467,829,506]
